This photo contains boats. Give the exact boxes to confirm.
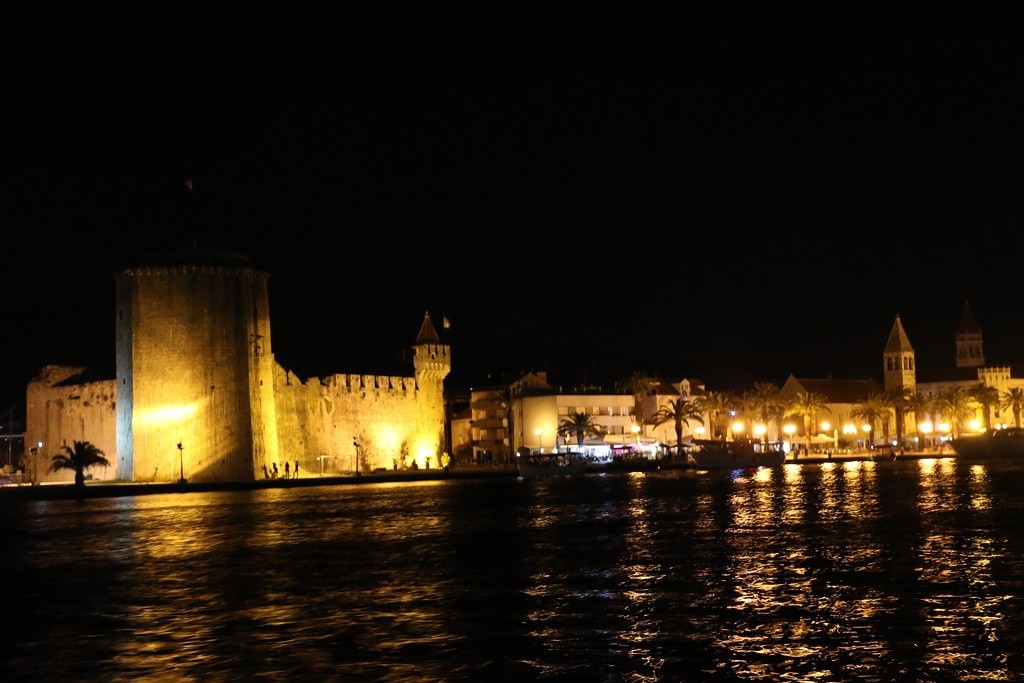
[948,427,1024,458]
[516,452,583,476]
[688,439,786,469]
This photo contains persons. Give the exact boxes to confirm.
[285,461,290,479]
[292,460,299,478]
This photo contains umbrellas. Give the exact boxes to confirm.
[791,433,836,449]
